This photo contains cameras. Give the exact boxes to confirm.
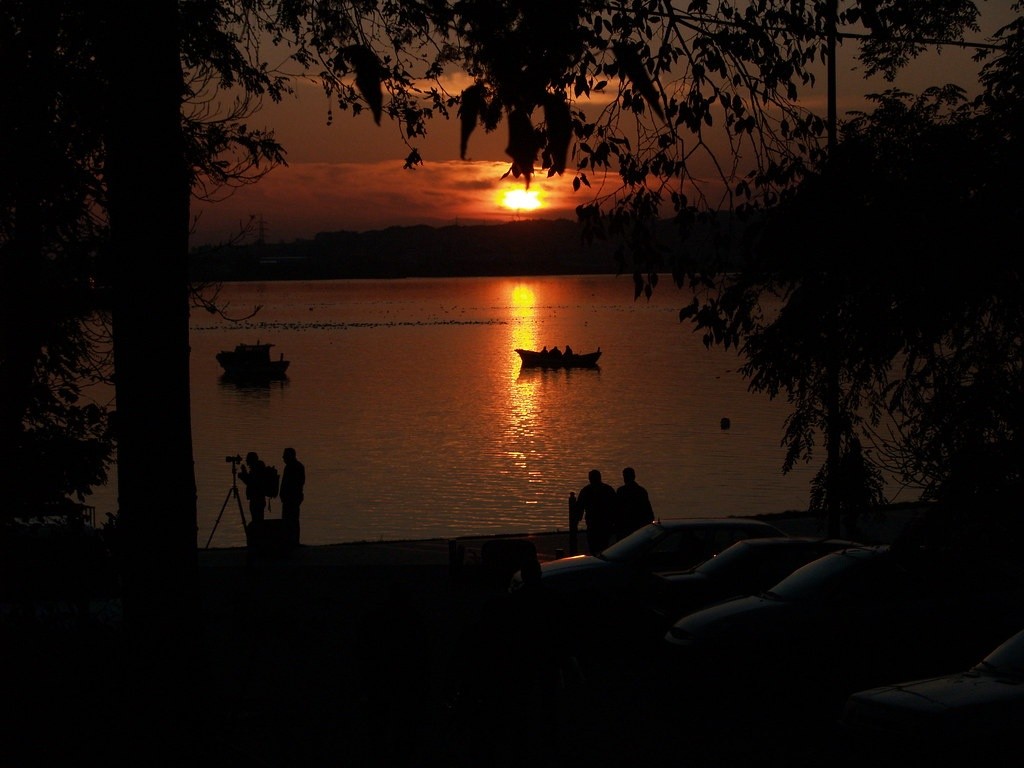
[226,453,243,464]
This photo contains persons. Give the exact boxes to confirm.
[571,470,619,552]
[280,448,308,547]
[541,343,575,359]
[237,451,266,520]
[616,468,655,538]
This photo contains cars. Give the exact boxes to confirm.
[505,515,1023,767]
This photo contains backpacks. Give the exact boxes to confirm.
[265,465,280,497]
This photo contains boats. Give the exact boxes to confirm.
[515,347,602,368]
[215,338,290,378]
